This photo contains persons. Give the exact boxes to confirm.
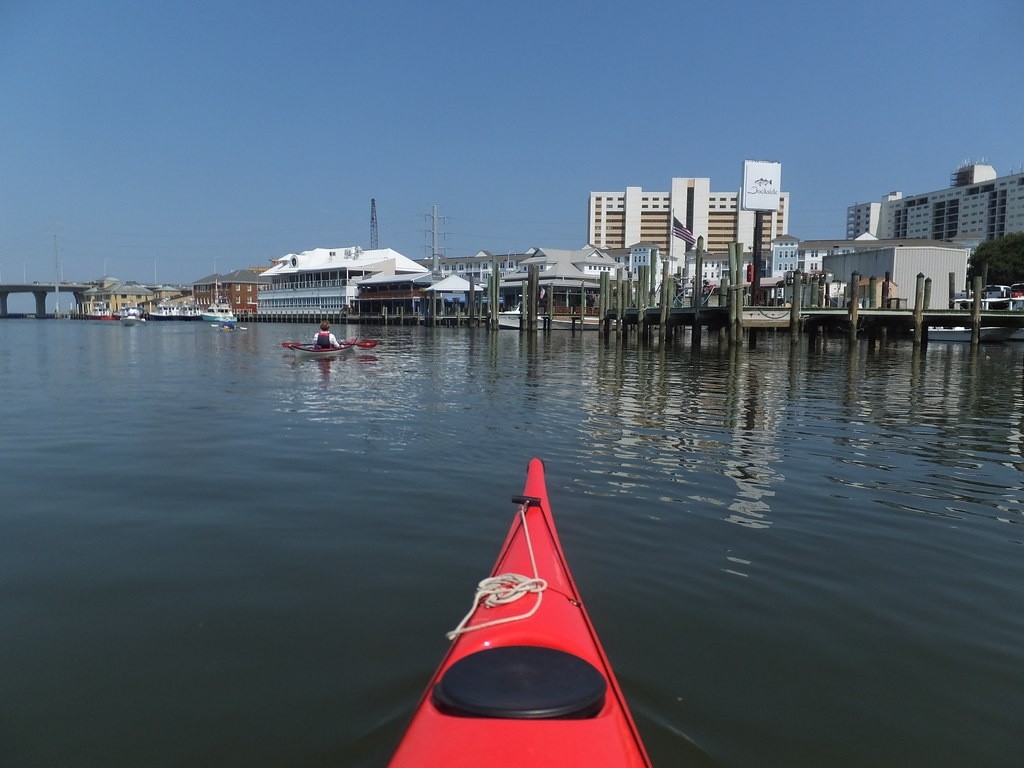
[222,317,234,329]
[312,320,344,349]
[703,280,710,288]
[690,277,695,287]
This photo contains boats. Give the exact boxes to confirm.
[201,278,237,322]
[120,307,147,326]
[281,335,360,355]
[85,300,120,320]
[149,300,203,322]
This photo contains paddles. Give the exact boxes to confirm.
[282,339,378,348]
[211,324,248,330]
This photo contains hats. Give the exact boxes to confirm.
[225,318,230,322]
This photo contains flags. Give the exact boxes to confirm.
[672,216,696,245]
